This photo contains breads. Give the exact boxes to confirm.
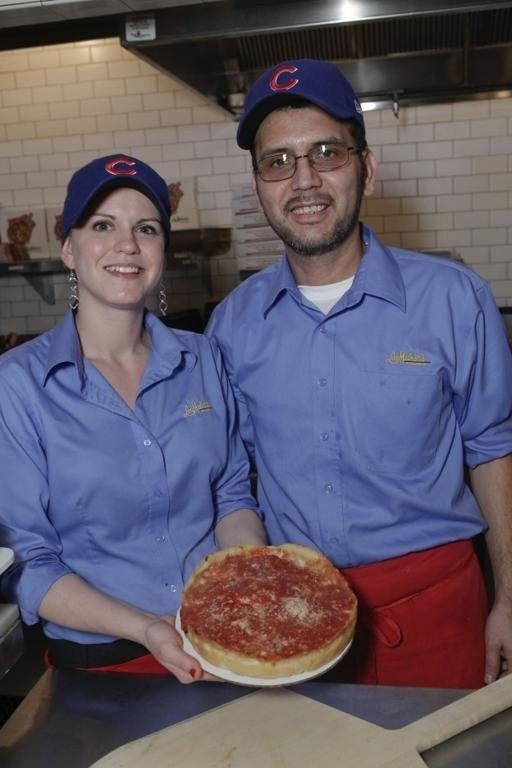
[182,544,359,679]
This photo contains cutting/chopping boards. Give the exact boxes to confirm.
[91,667,512,768]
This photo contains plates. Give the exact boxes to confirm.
[174,602,355,689]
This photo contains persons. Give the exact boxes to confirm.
[196,60,512,691]
[0,152,272,690]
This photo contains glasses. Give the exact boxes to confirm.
[254,144,355,182]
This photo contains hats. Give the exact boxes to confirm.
[233,57,368,153]
[60,153,172,252]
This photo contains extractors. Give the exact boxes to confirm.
[114,0,511,119]
